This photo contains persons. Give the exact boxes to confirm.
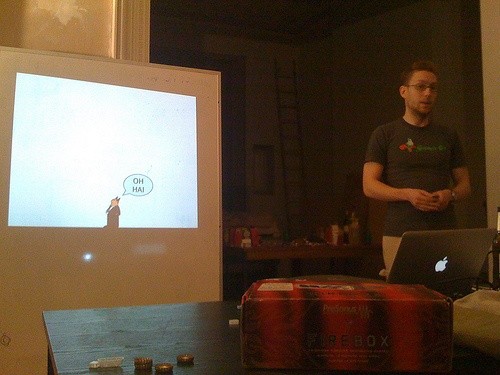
[361,59,473,283]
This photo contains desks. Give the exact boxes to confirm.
[42,280,500,375]
[223,247,364,280]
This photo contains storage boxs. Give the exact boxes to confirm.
[240,278,453,375]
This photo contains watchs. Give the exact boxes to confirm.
[448,188,458,203]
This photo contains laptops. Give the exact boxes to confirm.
[386,228,497,295]
